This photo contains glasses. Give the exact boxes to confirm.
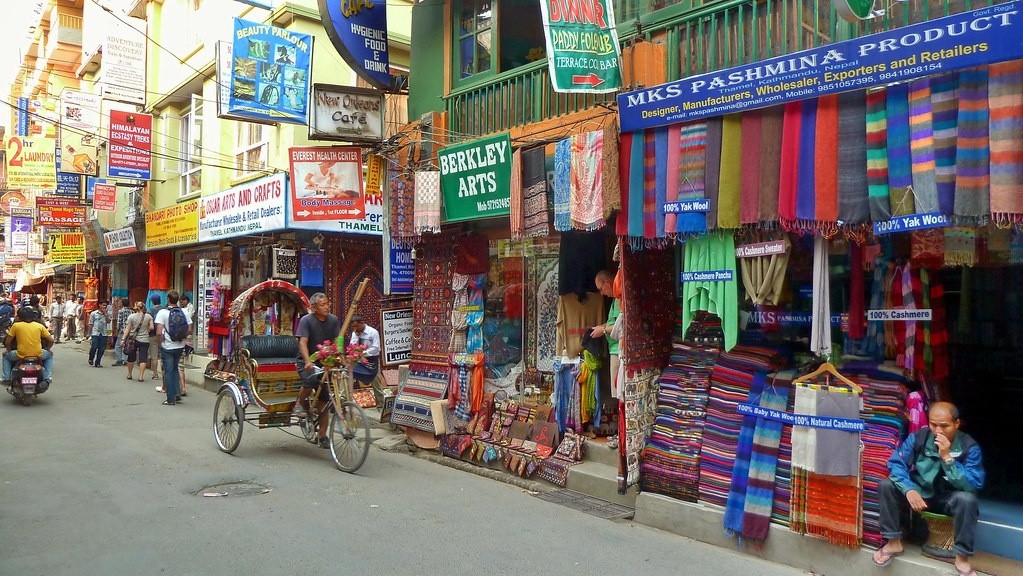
[599,282,605,292]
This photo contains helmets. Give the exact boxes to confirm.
[0,298,7,305]
[21,297,30,306]
[27,306,39,318]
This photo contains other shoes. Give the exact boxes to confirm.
[0,380,11,386]
[156,386,167,393]
[112,362,122,366]
[176,396,180,401]
[89,359,93,365]
[606,434,618,441]
[96,364,103,368]
[138,379,144,382]
[162,400,175,405]
[127,375,132,380]
[607,439,619,448]
[152,374,158,380]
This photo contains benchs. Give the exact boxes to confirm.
[242,334,303,399]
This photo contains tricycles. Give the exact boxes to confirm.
[203,278,370,474]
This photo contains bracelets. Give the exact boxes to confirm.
[602,324,607,334]
[105,314,107,317]
[121,338,126,341]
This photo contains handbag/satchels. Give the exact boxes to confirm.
[124,332,137,355]
[457,400,586,478]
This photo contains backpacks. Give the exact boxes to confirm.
[164,306,188,341]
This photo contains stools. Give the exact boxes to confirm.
[918,511,956,558]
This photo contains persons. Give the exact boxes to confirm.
[258,86,280,106]
[153,289,197,405]
[0,292,55,387]
[74,296,87,344]
[871,402,985,575]
[305,161,337,190]
[85,300,111,368]
[294,293,346,449]
[589,271,622,450]
[49,294,79,343]
[347,316,382,391]
[111,294,165,382]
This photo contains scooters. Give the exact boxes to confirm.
[6,356,46,406]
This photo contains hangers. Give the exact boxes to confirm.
[791,354,864,393]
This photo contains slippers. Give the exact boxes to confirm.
[315,437,330,448]
[872,547,904,567]
[293,405,308,418]
[954,565,978,576]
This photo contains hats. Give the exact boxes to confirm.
[151,294,160,301]
